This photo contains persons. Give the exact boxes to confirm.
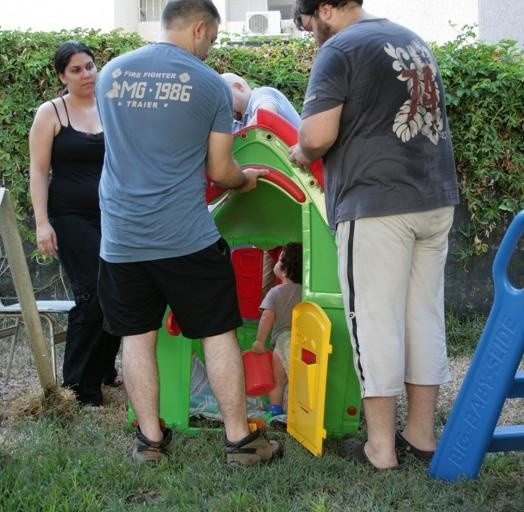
[27,40,124,408]
[95,1,282,468]
[288,1,459,469]
[218,73,304,134]
[253,242,300,416]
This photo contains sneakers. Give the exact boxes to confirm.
[133,424,172,464]
[222,424,284,469]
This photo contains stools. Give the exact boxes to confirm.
[1,300,75,389]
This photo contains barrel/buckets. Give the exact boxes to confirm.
[242,348,277,396]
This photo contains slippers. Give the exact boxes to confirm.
[338,438,405,469]
[396,430,436,460]
